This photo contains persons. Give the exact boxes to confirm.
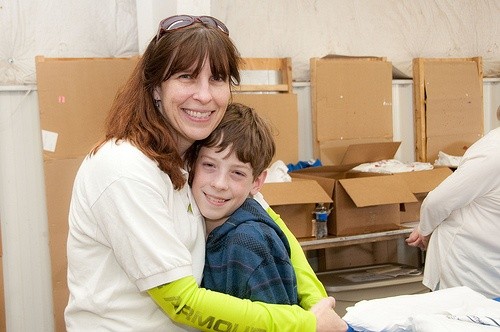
[63,15,348,332]
[405,103,500,300]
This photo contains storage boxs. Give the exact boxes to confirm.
[258,142,454,271]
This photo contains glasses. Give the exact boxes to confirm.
[157,15,229,40]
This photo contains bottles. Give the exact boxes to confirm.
[315,202,327,239]
